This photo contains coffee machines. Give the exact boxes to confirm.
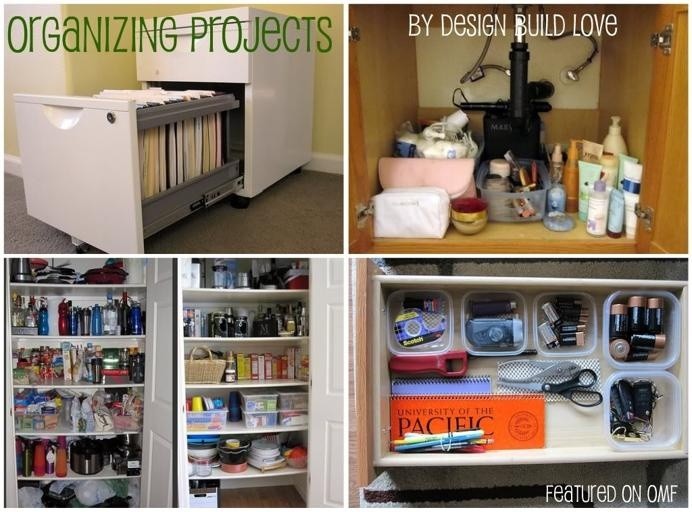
[112,433,140,475]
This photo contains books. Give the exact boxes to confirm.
[93,89,223,202]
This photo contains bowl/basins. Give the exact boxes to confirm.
[37,266,128,284]
[189,436,250,474]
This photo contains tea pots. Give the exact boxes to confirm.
[211,265,234,290]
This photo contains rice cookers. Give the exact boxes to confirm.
[71,438,103,473]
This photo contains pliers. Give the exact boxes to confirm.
[498,360,581,383]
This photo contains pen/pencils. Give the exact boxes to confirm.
[389,428,494,453]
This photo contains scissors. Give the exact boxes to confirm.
[496,369,603,407]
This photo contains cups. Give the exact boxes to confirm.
[214,259,224,266]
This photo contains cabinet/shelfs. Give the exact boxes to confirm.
[174,257,347,508]
[5,258,174,508]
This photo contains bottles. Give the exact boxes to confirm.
[13,291,144,431]
[238,258,308,289]
[181,302,309,335]
[549,144,563,183]
[11,259,33,281]
[16,438,67,478]
[548,181,566,211]
[586,180,607,236]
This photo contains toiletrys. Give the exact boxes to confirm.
[208,301,307,337]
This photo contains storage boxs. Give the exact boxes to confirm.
[386,288,683,454]
[276,407,308,426]
[238,389,279,412]
[239,404,278,428]
[475,158,551,224]
[185,405,231,431]
[272,388,308,409]
